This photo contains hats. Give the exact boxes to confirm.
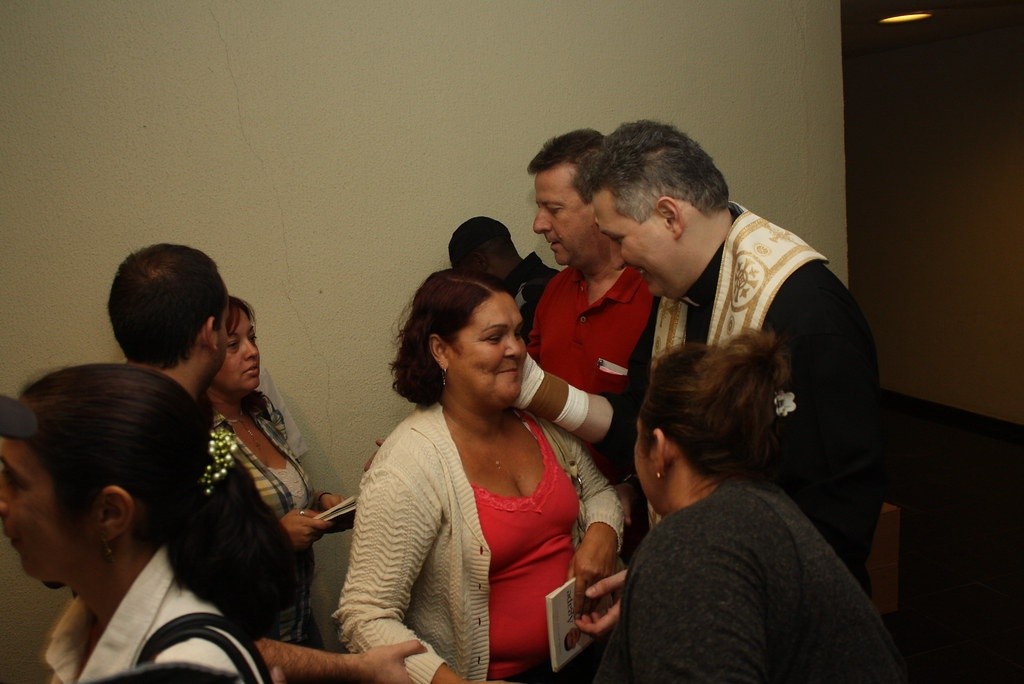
[448,216,511,270]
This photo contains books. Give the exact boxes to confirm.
[312,496,358,521]
[545,577,614,671]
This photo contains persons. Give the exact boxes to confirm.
[364,128,653,567]
[449,216,559,342]
[511,119,890,595]
[0,363,288,684]
[208,296,346,652]
[564,593,614,650]
[107,244,427,684]
[594,330,907,684]
[330,269,625,684]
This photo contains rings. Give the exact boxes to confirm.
[299,508,304,515]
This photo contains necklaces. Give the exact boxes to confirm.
[449,422,510,470]
[234,416,261,449]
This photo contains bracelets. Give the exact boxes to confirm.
[620,474,646,509]
[318,492,332,510]
